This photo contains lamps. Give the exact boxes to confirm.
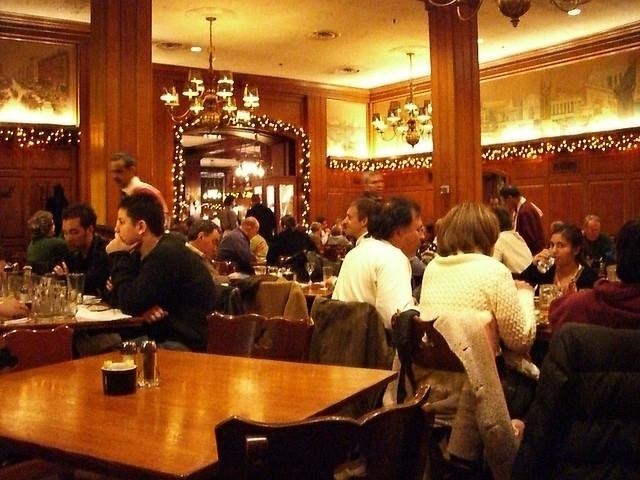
[371,50,432,148]
[428,0,582,28]
[159,17,261,125]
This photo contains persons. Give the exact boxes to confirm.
[43,184,69,237]
[490,186,640,330]
[332,195,436,330]
[246,194,277,240]
[176,214,356,284]
[0,296,28,321]
[26,210,71,274]
[419,201,538,378]
[106,188,216,352]
[219,194,237,230]
[177,221,231,315]
[110,152,171,242]
[51,203,114,293]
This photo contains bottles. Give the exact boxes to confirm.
[141,340,160,389]
[120,341,137,365]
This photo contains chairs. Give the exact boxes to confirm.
[391,309,504,478]
[254,280,310,319]
[204,311,315,363]
[214,384,432,479]
[1,323,75,375]
[311,297,391,372]
[325,242,347,260]
[514,323,640,479]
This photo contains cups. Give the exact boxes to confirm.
[537,257,556,273]
[100,366,138,396]
[536,281,577,323]
[252,266,334,291]
[1,261,85,325]
